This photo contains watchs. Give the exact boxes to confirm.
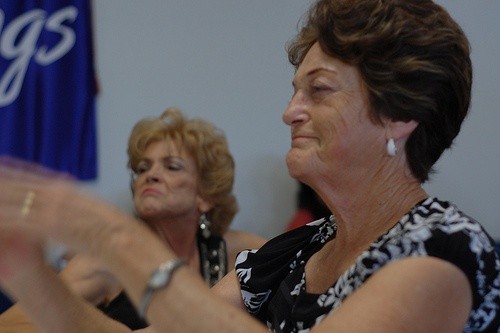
[137,254,189,321]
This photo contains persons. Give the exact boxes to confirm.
[0,1,500,333]
[0,109,272,332]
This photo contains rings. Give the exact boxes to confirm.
[18,191,36,219]
